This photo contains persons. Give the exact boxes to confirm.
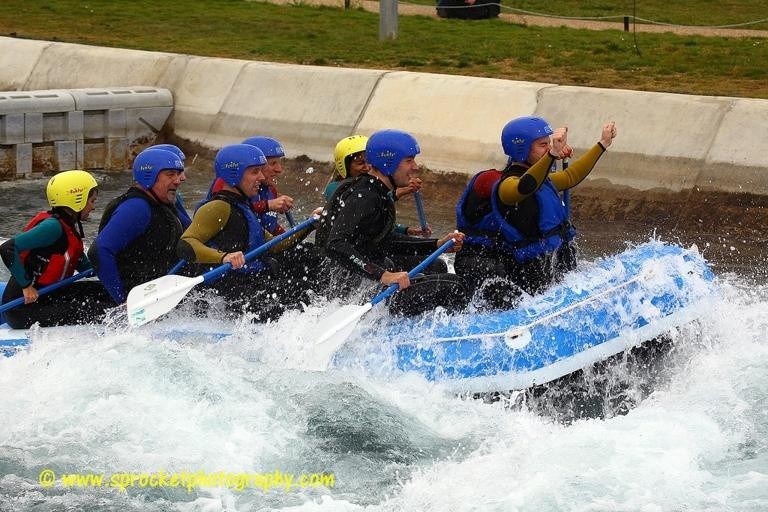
[327,130,471,318]
[0,170,117,330]
[322,135,431,259]
[453,144,575,293]
[492,117,618,302]
[243,138,314,268]
[176,144,328,322]
[144,144,222,250]
[87,148,198,325]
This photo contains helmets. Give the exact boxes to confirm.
[335,135,369,179]
[46,169,98,213]
[242,136,284,157]
[147,143,186,161]
[366,129,419,176]
[214,143,268,186]
[502,117,553,163]
[134,149,183,191]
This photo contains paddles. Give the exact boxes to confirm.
[126,214,322,328]
[310,229,462,356]
